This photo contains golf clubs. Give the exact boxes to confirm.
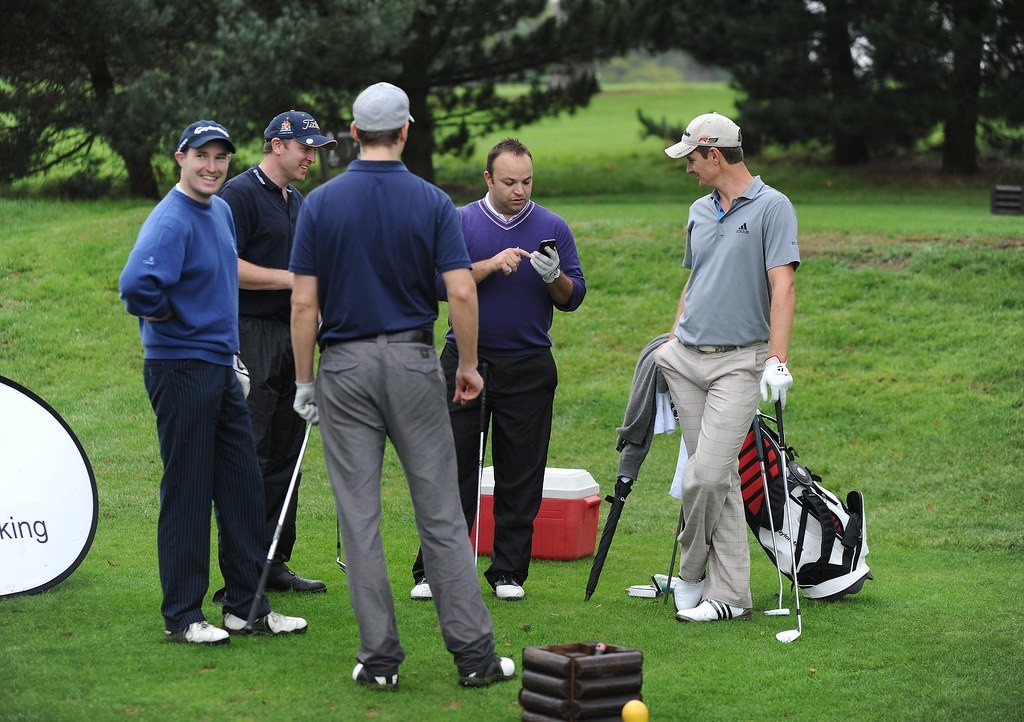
[336,509,346,573]
[244,422,313,634]
[753,414,791,615]
[775,393,802,644]
[474,361,489,567]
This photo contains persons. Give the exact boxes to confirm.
[118,120,308,645]
[215,109,339,599]
[653,111,801,623]
[409,138,587,601]
[288,81,516,690]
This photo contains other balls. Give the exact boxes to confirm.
[622,700,649,722]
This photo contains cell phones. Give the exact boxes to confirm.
[538,238,556,259]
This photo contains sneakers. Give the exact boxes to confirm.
[491,576,525,600]
[457,656,515,684]
[265,567,328,594]
[164,620,229,646]
[409,574,433,600]
[351,660,400,691]
[223,606,308,635]
[676,601,752,625]
[673,568,706,611]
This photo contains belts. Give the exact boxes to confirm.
[363,328,434,345]
[695,343,745,355]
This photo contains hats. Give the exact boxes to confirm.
[264,110,338,151]
[664,112,742,159]
[352,81,415,131]
[177,120,236,155]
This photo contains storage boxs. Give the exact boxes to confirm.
[470,466,602,559]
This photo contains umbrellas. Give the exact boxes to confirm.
[583,476,636,602]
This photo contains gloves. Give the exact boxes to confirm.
[293,380,319,426]
[530,245,562,284]
[232,354,251,400]
[760,356,794,410]
